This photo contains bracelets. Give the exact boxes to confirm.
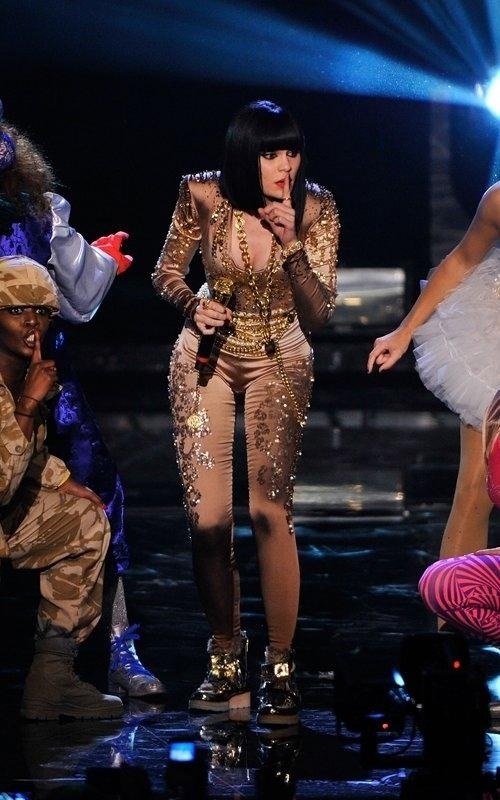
[280,240,304,261]
[13,410,34,418]
[19,395,40,406]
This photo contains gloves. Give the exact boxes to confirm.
[89,231,134,276]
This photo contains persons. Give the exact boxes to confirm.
[1,122,166,699]
[1,254,123,724]
[418,395,498,646]
[151,97,343,728]
[363,181,499,650]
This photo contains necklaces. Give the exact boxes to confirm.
[234,200,278,360]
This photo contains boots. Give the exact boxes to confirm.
[19,635,126,722]
[108,622,167,698]
[255,644,303,728]
[188,632,252,713]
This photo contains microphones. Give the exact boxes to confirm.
[196,277,233,372]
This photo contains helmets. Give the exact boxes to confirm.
[1,255,62,317]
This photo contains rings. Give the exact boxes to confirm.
[202,299,213,310]
[284,197,292,201]
